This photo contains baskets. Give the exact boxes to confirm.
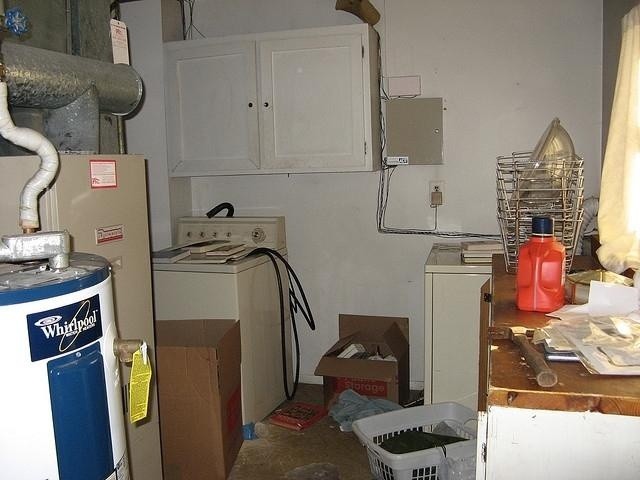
[352,402,477,479]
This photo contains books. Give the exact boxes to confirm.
[269,402,328,430]
[543,338,580,362]
[337,343,366,359]
[152,240,257,263]
[461,241,505,264]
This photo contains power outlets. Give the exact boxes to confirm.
[429,182,445,205]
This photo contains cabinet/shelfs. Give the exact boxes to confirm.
[164,22,384,180]
[477,253,640,480]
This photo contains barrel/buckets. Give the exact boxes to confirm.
[514,215,567,312]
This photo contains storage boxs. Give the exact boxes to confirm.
[315,313,410,411]
[156,318,242,480]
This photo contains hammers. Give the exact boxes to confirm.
[489,326,558,388]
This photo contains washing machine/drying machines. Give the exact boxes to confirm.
[152,216,295,427]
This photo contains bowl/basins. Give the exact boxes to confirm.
[515,116,574,204]
